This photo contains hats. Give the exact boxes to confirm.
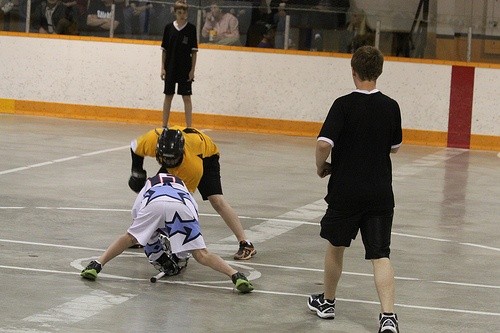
[262,24,276,34]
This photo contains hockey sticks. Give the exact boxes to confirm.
[148,267,166,284]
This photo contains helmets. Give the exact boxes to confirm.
[156,128,185,168]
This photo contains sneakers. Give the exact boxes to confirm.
[378,312,399,333]
[80,260,102,280]
[234,241,257,260]
[128,243,145,248]
[307,293,336,319]
[232,272,254,293]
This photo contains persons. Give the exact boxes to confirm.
[79,172,255,294]
[200,4,241,46]
[306,45,404,333]
[128,126,257,262]
[246,0,351,49]
[0,0,21,15]
[159,0,198,129]
[32,0,152,38]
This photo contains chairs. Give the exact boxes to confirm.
[1,0,354,55]
